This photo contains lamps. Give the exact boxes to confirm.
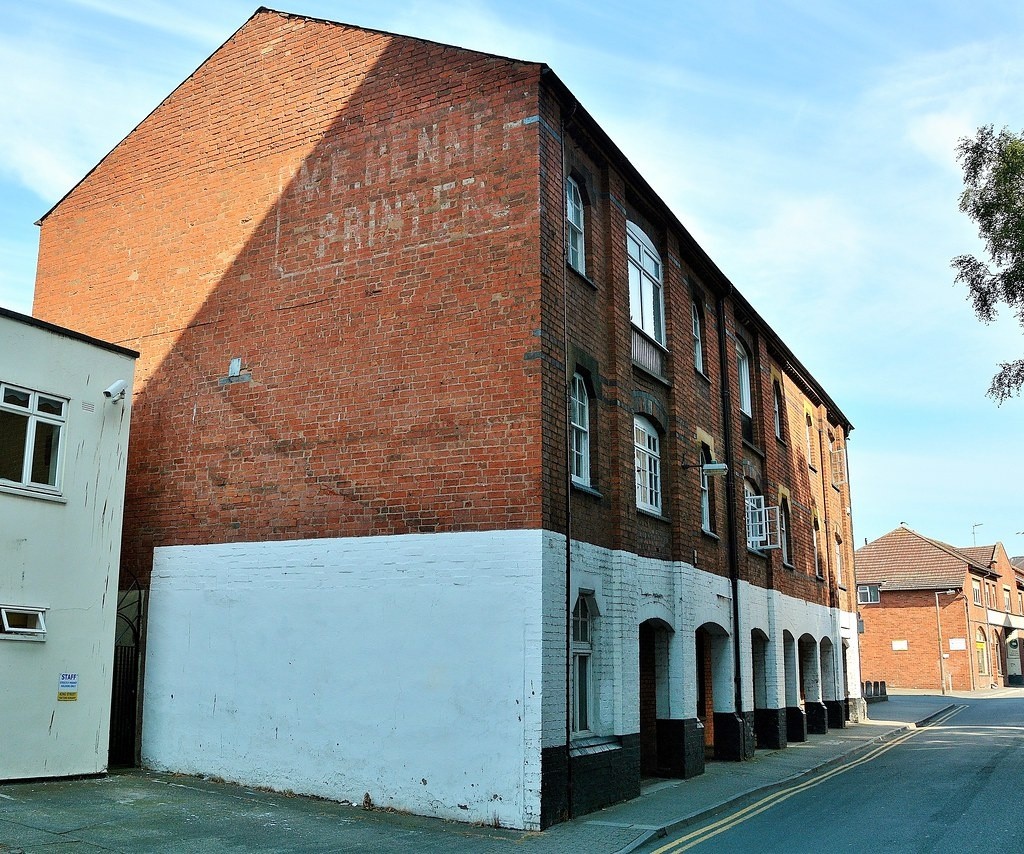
[682,463,728,476]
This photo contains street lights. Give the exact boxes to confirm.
[683,461,730,479]
[935,590,955,695]
[970,523,986,546]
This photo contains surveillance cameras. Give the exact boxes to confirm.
[103,380,128,398]
